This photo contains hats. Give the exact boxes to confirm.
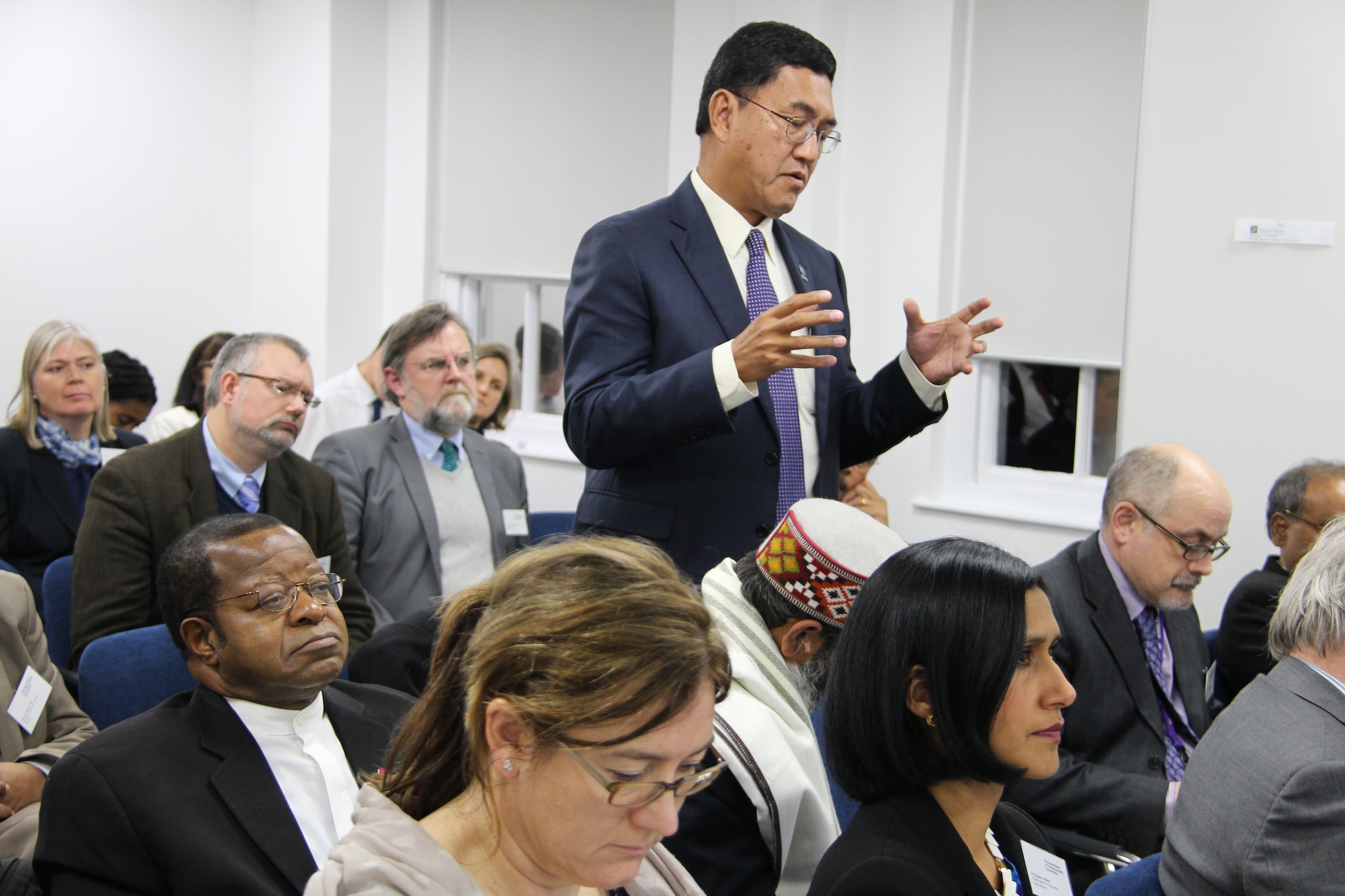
[756,497,911,629]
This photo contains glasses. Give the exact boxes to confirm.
[556,738,726,811]
[238,371,320,408]
[179,572,344,621]
[731,92,841,153]
[403,352,478,373]
[1134,504,1230,562]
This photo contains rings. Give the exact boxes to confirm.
[862,498,867,505]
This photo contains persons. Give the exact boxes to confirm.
[0,304,1076,896]
[562,23,1003,593]
[512,321,564,417]
[1218,459,1345,707]
[1158,515,1345,896]
[997,442,1233,859]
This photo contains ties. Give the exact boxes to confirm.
[1134,604,1187,783]
[440,439,458,470]
[746,229,807,527]
[239,474,261,514]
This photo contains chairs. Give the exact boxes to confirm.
[77,624,197,731]
